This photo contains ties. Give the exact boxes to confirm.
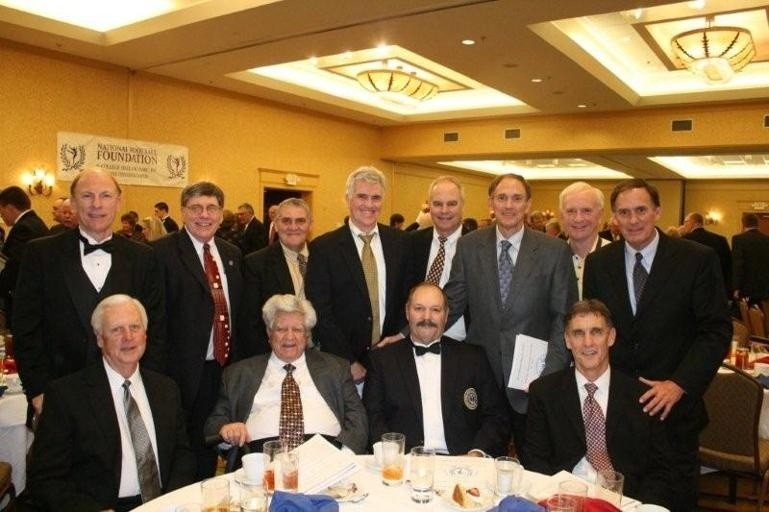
[412,341,441,356]
[425,232,448,286]
[279,364,304,453]
[576,258,584,302]
[358,233,380,347]
[583,383,616,489]
[297,253,307,280]
[269,224,276,246]
[203,242,231,366]
[633,252,649,307]
[122,380,162,504]
[497,240,515,310]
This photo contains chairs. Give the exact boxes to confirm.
[689,361,764,511]
[731,315,752,347]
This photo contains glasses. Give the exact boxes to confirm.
[237,212,247,216]
[183,205,223,214]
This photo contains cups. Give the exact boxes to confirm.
[410,445,436,502]
[273,449,301,493]
[558,478,589,511]
[494,454,521,498]
[237,476,269,512]
[198,475,232,512]
[380,431,406,488]
[545,495,579,511]
[372,440,398,469]
[594,469,625,509]
[242,453,272,480]
[261,439,282,462]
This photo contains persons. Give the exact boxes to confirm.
[379,175,579,453]
[399,175,474,344]
[241,197,317,354]
[598,215,622,240]
[47,196,66,233]
[731,214,769,304]
[60,196,80,230]
[112,201,179,245]
[1,186,49,282]
[151,181,246,481]
[217,202,279,250]
[679,209,730,290]
[453,209,496,236]
[27,294,192,512]
[518,300,699,512]
[582,178,731,511]
[558,180,613,301]
[213,294,366,469]
[303,165,415,383]
[5,170,158,425]
[361,284,512,456]
[385,211,405,231]
[528,205,567,239]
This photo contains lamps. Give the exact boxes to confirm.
[503,158,597,171]
[628,6,765,84]
[702,156,763,170]
[313,56,473,112]
[18,165,57,199]
[701,208,725,228]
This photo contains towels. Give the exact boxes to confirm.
[486,495,545,512]
[268,490,339,512]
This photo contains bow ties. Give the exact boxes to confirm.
[80,235,116,255]
